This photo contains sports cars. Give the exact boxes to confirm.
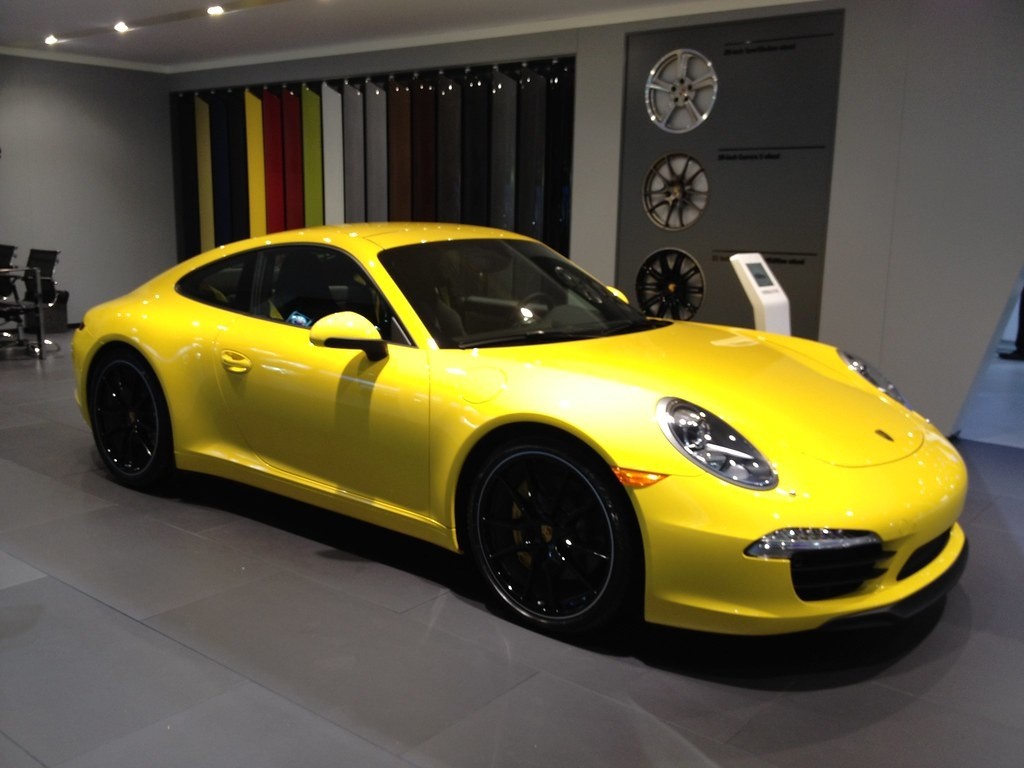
[70,222,969,645]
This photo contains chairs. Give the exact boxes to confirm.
[0,248,61,356]
[435,262,491,335]
[0,243,25,341]
